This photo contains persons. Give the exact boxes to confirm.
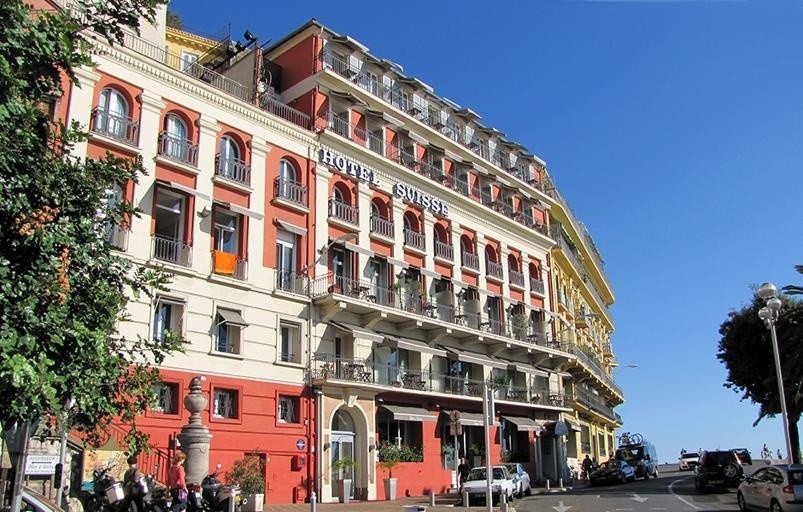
[698,447,703,454]
[458,458,471,495]
[119,457,143,512]
[168,452,188,512]
[608,453,614,461]
[61,485,68,508]
[591,457,598,471]
[681,448,686,455]
[582,455,592,479]
[777,449,782,459]
[763,443,768,452]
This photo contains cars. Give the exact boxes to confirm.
[736,463,802,512]
[498,462,532,497]
[590,460,637,484]
[678,452,701,471]
[730,449,752,465]
[460,465,517,504]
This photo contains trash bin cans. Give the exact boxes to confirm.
[786,463,803,500]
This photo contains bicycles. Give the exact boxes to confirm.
[617,432,644,446]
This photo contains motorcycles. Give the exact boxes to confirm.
[82,461,138,511]
[140,464,188,512]
[201,464,248,512]
[186,480,211,512]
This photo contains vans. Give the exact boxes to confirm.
[615,441,660,480]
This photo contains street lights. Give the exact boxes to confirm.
[756,282,792,465]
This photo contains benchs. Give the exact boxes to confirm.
[353,286,561,350]
[342,363,565,406]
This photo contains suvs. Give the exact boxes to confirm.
[693,449,745,492]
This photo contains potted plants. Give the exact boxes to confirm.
[239,463,264,511]
[330,456,360,503]
[376,444,402,499]
[320,363,333,378]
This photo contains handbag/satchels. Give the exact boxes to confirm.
[177,488,188,504]
